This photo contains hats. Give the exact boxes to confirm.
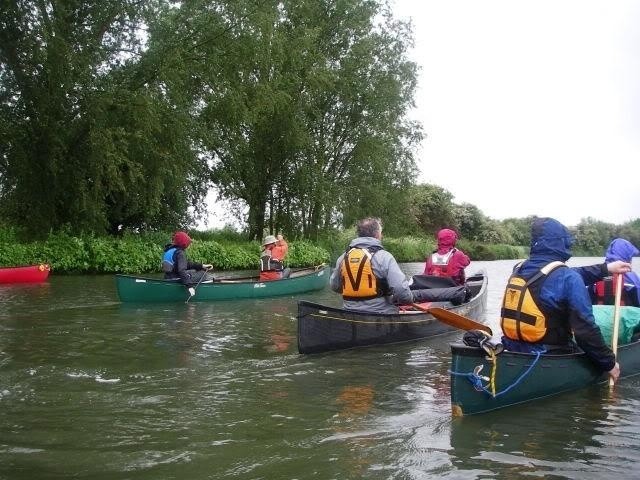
[263,236,278,246]
[174,231,192,248]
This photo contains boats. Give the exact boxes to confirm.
[451,343,640,418]
[116,265,330,302]
[299,274,488,354]
[0,263,50,285]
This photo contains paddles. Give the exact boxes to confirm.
[410,303,493,337]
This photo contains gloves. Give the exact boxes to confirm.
[203,264,213,270]
[189,287,195,296]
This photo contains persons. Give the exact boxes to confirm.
[259,235,291,281]
[500,219,631,380]
[330,217,412,314]
[584,236,640,307]
[424,229,469,284]
[161,231,213,296]
